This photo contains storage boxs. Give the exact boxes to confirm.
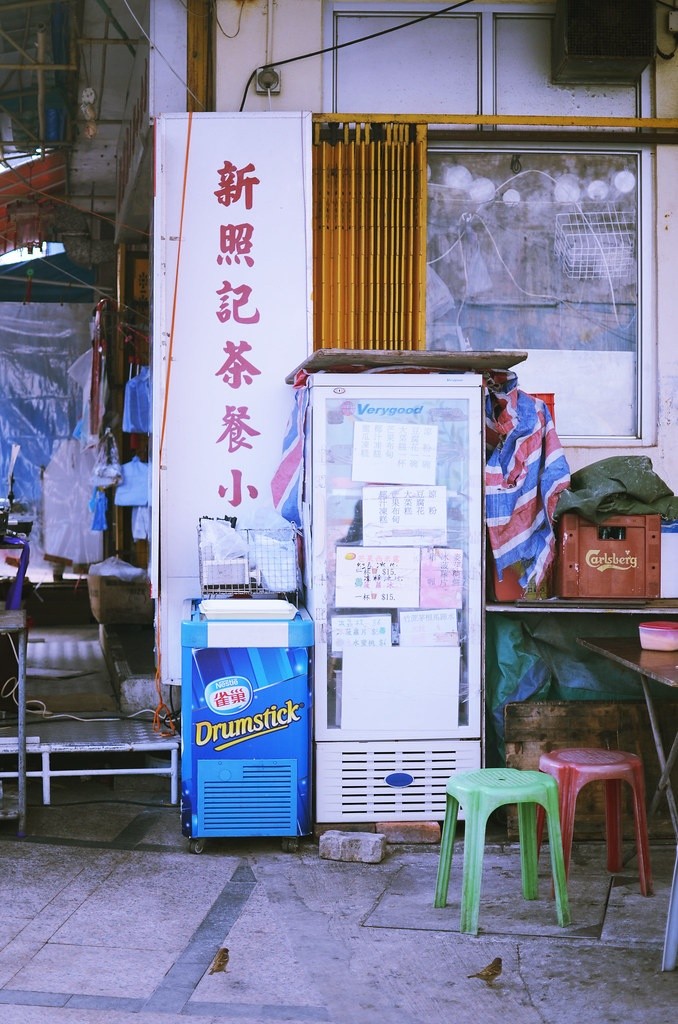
[554,513,678,598]
[87,576,151,625]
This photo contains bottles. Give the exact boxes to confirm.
[602,526,625,539]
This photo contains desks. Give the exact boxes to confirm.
[578,637,678,866]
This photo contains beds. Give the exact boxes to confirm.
[0,711,181,806]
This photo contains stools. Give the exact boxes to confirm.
[435,767,572,934]
[532,748,653,897]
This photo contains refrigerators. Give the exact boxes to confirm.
[179,596,316,855]
[304,372,489,824]
